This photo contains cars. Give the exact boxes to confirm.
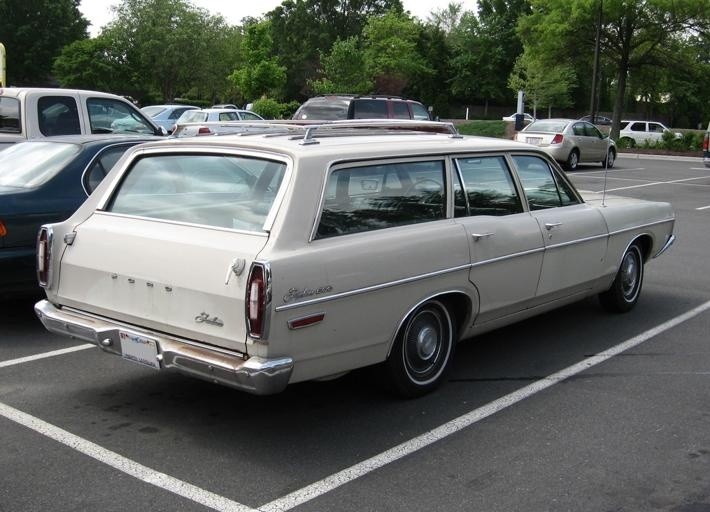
[502,113,537,121]
[702,121,710,168]
[513,118,617,170]
[609,120,683,149]
[579,116,612,125]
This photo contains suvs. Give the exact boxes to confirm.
[32,119,674,399]
